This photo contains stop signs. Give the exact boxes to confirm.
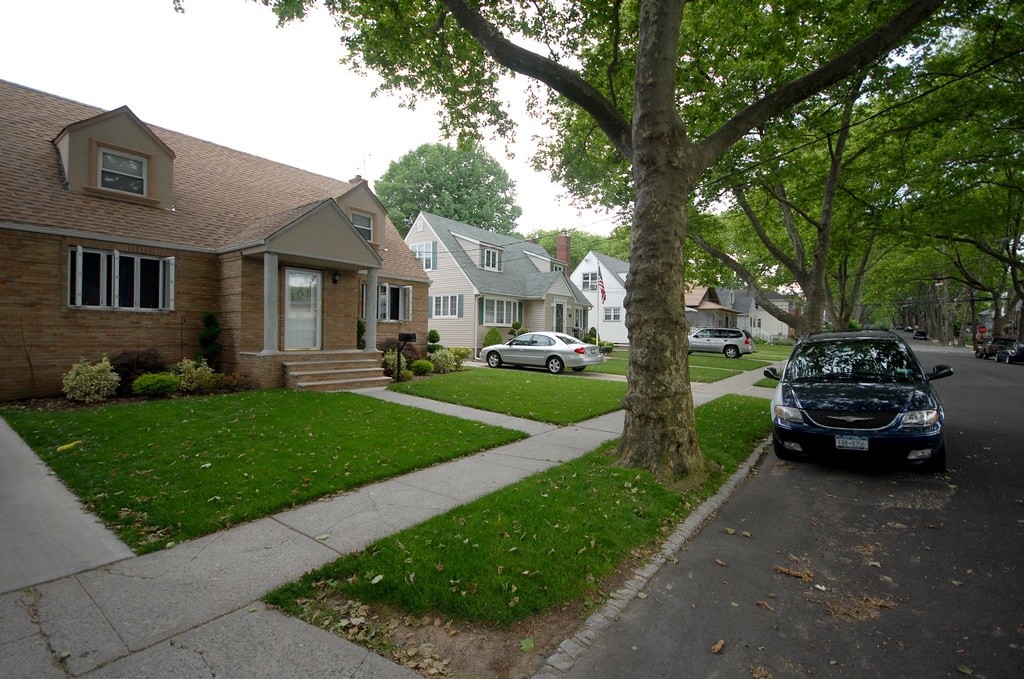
[978,326,987,333]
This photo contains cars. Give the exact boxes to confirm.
[478,330,604,374]
[896,325,928,340]
[762,327,955,470]
[974,332,1024,364]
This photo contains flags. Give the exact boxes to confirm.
[599,266,606,304]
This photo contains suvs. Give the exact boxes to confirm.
[688,327,759,358]
